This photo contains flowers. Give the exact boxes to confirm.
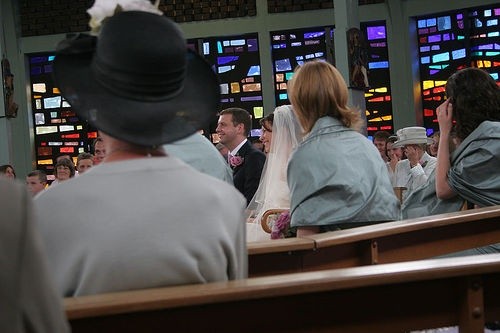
[270,211,298,239]
[87,0,163,38]
[420,160,428,167]
[229,156,244,168]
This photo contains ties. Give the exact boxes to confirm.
[228,155,234,171]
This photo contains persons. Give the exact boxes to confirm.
[286,60,400,237]
[0,0,500,333]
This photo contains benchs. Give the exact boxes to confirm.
[61,186,500,333]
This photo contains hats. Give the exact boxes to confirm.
[391,127,435,148]
[52,10,222,146]
